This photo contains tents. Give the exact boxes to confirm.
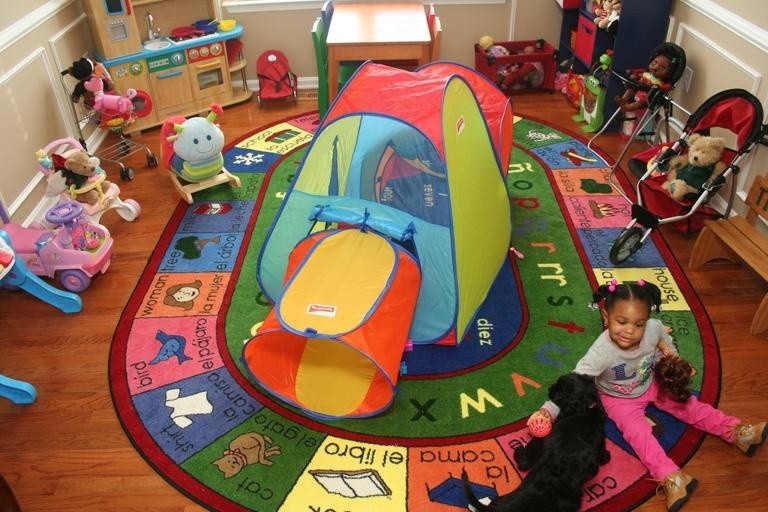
[240,54,513,423]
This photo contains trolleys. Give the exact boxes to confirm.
[59,63,158,182]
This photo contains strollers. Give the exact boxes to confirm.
[608,87,767,265]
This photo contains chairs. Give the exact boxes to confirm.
[690,174,768,334]
[313,1,441,119]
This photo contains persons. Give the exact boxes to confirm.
[527,275,768,512]
[613,48,676,113]
[653,352,696,404]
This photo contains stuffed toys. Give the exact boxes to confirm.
[61,56,140,134]
[478,27,559,95]
[160,102,246,206]
[661,131,729,200]
[555,0,623,137]
[57,150,111,204]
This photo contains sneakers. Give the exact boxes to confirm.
[644,469,698,512]
[720,418,768,458]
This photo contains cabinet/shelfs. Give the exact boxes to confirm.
[107,38,251,138]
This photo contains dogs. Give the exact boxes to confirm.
[461,373,613,511]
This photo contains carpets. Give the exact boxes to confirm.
[107,102,721,510]
